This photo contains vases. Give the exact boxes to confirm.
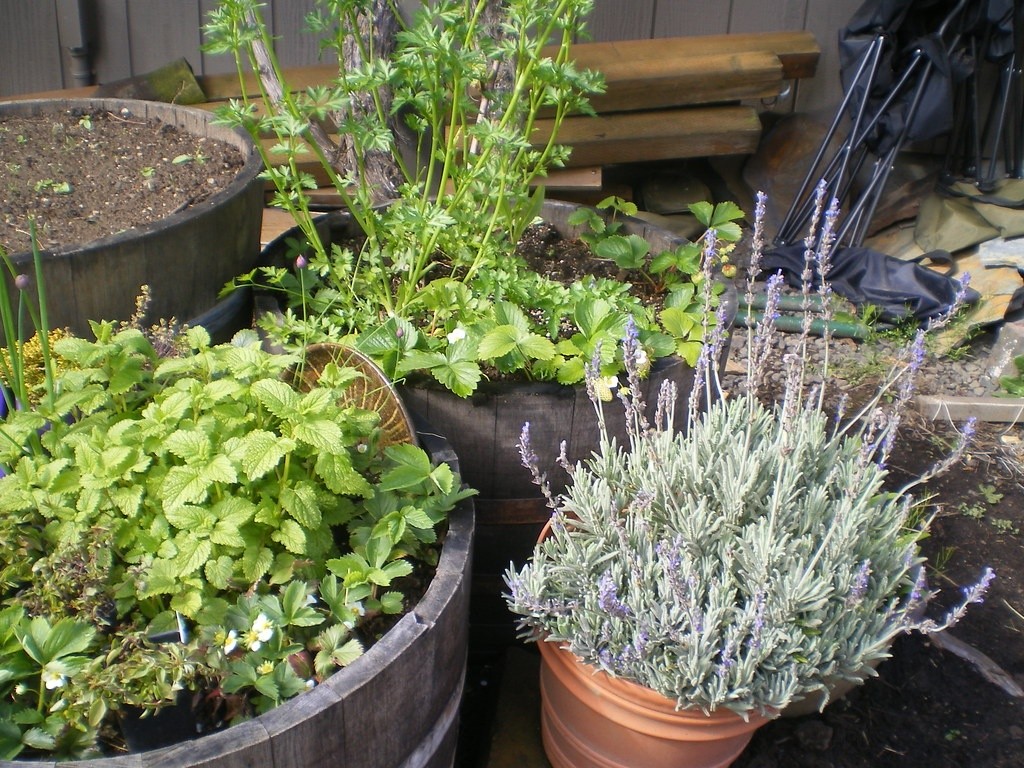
[531,508,893,768]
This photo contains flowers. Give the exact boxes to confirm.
[501,180,999,721]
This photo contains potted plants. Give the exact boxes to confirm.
[0,212,480,768]
[201,1,737,632]
[0,98,265,352]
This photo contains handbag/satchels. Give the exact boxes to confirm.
[743,236,983,335]
